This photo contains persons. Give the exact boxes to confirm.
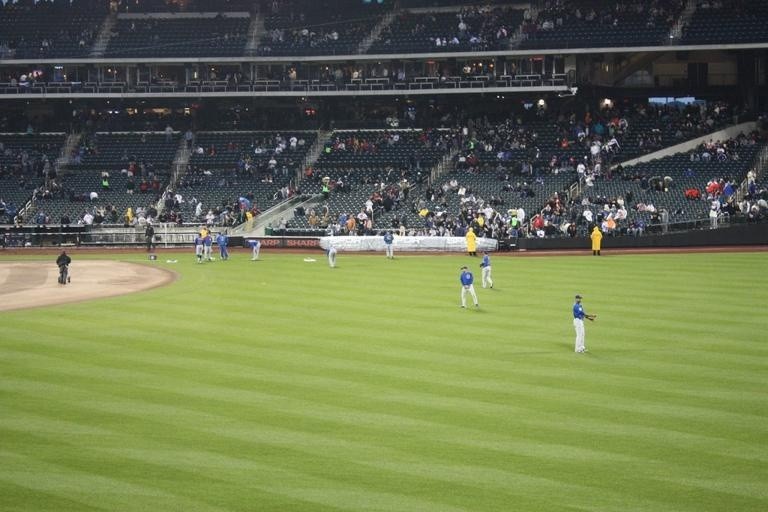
[459,266,476,308]
[573,295,596,354]
[1,2,768,269]
[56,250,71,285]
[479,251,493,289]
